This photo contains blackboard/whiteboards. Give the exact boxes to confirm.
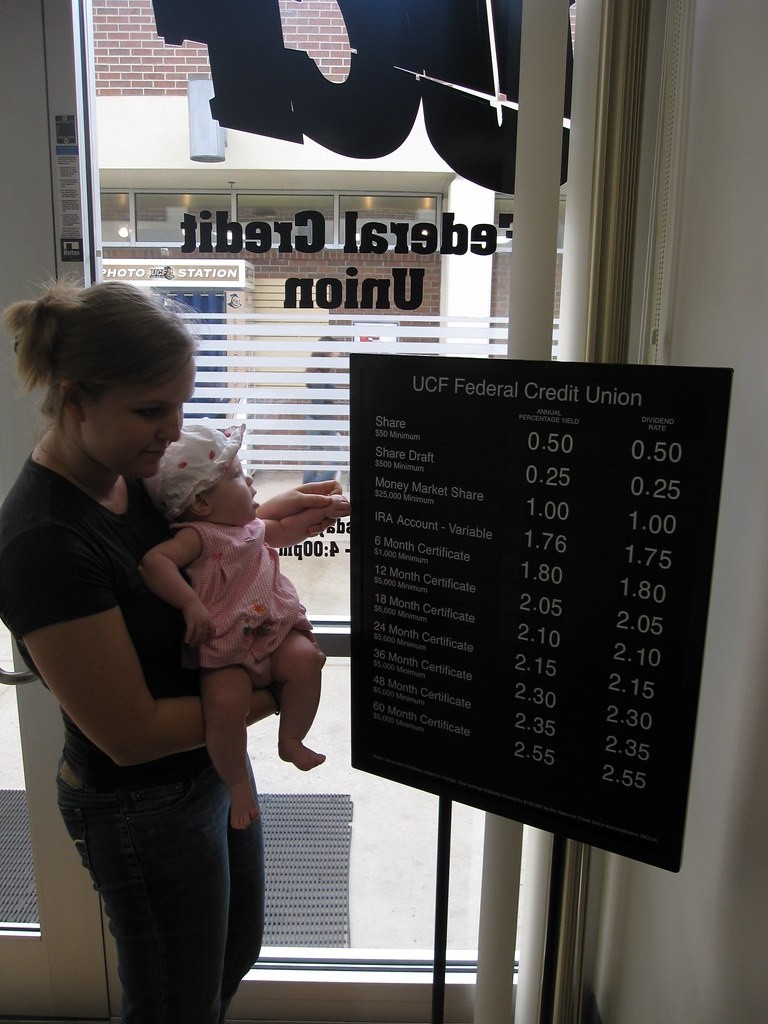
[349,355,732,875]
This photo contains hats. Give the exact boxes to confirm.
[142,423,247,524]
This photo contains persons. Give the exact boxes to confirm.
[0,281,340,1024]
[139,425,349,827]
[300,334,339,484]
